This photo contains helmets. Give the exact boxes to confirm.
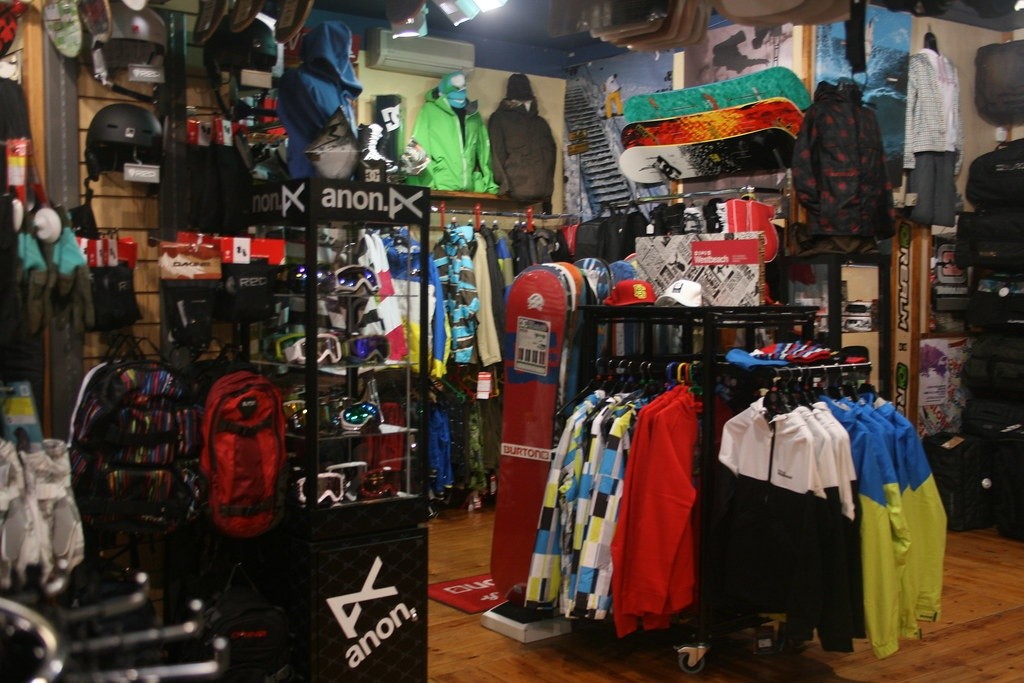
[202,19,276,70]
[84,103,162,181]
[92,2,166,53]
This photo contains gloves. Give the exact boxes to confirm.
[0,191,86,339]
[0,435,84,595]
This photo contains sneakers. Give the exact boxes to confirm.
[358,124,398,173]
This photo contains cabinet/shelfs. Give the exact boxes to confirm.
[162,181,431,683]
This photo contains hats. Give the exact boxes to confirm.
[656,279,703,307]
[604,278,655,306]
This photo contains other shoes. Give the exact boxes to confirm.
[649,200,728,233]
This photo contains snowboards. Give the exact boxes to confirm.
[488,256,638,596]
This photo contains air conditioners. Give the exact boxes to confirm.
[365,27,476,79]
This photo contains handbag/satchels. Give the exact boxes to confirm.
[959,335,1024,400]
[967,139,1024,207]
[954,212,1024,269]
[964,277,1024,332]
[960,400,1024,437]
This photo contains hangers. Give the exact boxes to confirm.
[556,357,704,429]
[446,209,567,238]
[762,362,879,422]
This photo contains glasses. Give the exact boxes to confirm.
[260,330,389,365]
[276,261,381,294]
[279,386,380,429]
[290,454,400,504]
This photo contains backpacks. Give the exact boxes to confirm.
[992,443,1024,540]
[68,335,290,539]
[921,432,991,530]
[575,201,645,263]
